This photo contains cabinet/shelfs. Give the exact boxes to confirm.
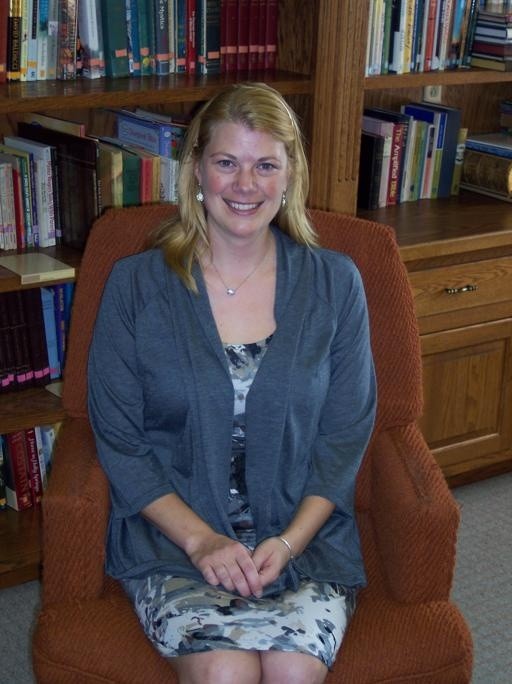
[334,0,511,491]
[1,1,343,591]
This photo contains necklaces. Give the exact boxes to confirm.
[204,242,269,293]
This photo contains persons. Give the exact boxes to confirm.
[86,80,380,683]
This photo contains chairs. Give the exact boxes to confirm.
[32,201,471,682]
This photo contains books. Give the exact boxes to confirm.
[363,1,511,77]
[2,1,281,81]
[1,421,65,511]
[355,0,512,212]
[357,99,511,211]
[1,283,79,397]
[3,106,196,251]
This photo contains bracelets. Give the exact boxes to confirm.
[276,536,296,562]
